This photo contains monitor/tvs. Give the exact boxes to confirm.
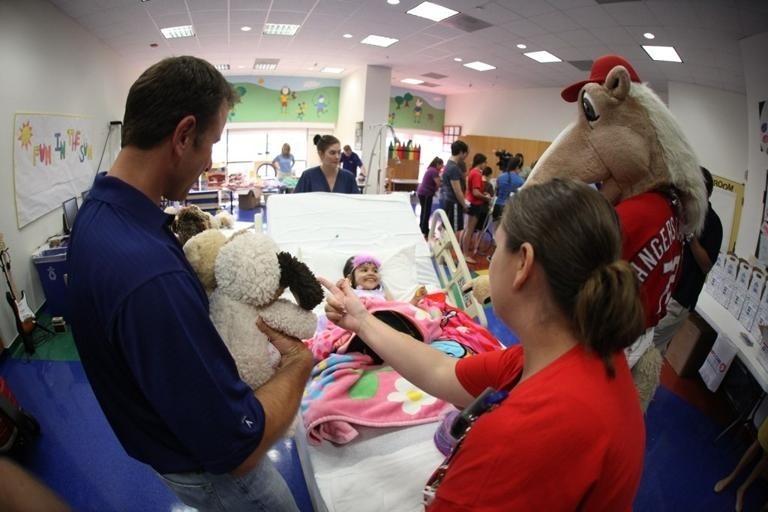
[62,196,79,233]
[82,190,89,201]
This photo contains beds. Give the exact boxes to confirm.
[255,191,488,512]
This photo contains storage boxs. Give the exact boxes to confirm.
[663,315,712,377]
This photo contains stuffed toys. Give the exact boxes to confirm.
[517,57,706,419]
[165,203,323,438]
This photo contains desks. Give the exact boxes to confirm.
[694,287,768,391]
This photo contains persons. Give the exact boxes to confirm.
[316,178,646,512]
[66,56,315,512]
[331,255,428,365]
[294,134,359,193]
[418,141,540,263]
[339,146,366,176]
[271,144,295,193]
[652,166,723,353]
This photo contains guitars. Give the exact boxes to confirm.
[0,234,37,335]
